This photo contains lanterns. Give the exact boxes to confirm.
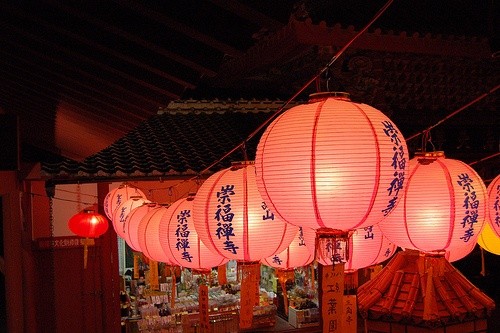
[371,233,397,266]
[111,195,153,237]
[138,204,175,265]
[262,226,314,272]
[377,148,489,255]
[122,202,162,253]
[105,184,147,221]
[404,238,477,263]
[255,92,408,239]
[193,162,296,265]
[159,192,231,273]
[487,173,500,237]
[302,225,381,275]
[67,209,109,269]
